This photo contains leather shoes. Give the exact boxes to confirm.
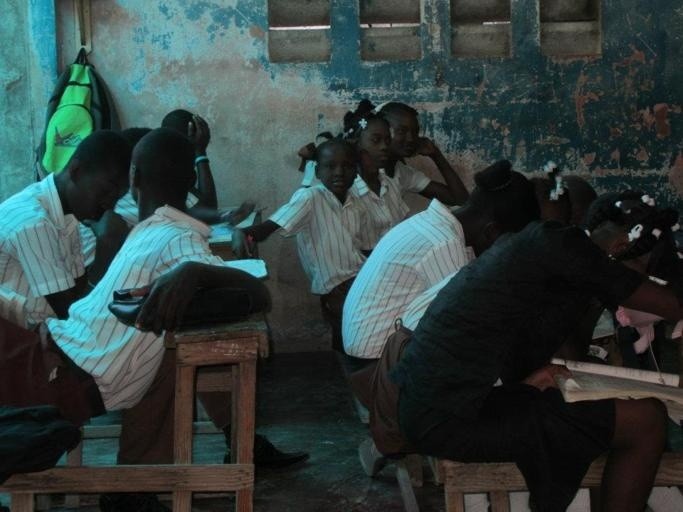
[224,434,309,470]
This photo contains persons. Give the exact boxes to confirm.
[228,103,683,511]
[1,108,308,512]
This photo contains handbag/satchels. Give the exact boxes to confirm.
[108,288,146,328]
[0,405,81,485]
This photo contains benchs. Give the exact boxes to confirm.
[0,206,270,512]
[422,457,683,511]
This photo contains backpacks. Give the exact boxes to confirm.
[368,318,416,460]
[34,48,122,181]
